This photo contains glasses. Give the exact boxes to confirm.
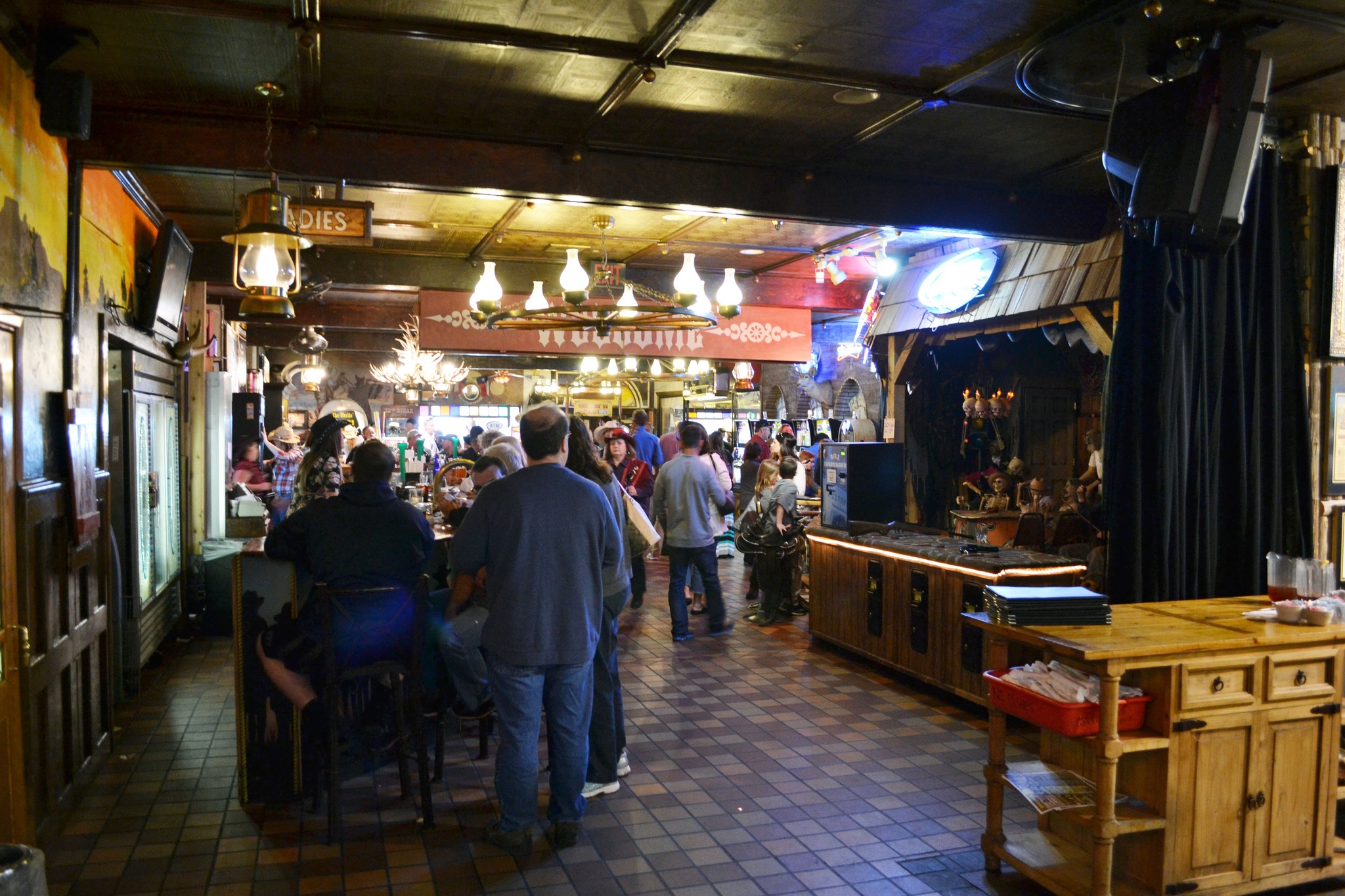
[806,459,814,464]
[470,441,477,445]
[471,487,481,495]
[565,431,572,439]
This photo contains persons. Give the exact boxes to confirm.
[257,442,435,757]
[443,403,625,848]
[650,425,736,641]
[232,400,827,635]
[430,455,510,722]
[240,590,294,756]
[1058,531,1108,584]
[285,413,350,517]
[602,428,653,609]
[1079,427,1104,498]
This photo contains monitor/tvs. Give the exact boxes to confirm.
[1102,46,1275,252]
[140,218,200,342]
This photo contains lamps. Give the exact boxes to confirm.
[815,261,827,283]
[221,81,314,321]
[825,256,847,286]
[301,327,325,391]
[875,241,896,275]
[369,214,755,406]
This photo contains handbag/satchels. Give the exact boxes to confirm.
[610,473,661,558]
[717,490,737,516]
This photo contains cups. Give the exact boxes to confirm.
[1296,558,1337,601]
[1306,606,1335,626]
[409,470,433,518]
[229,500,239,517]
[1266,551,1297,606]
[1274,601,1305,622]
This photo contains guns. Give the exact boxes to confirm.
[958,543,999,555]
[846,518,976,539]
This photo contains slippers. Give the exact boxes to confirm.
[685,598,710,615]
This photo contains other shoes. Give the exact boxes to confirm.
[549,822,580,845]
[790,604,806,615]
[581,780,621,796]
[744,559,753,565]
[647,553,660,560]
[779,603,791,612]
[479,822,532,854]
[451,700,496,720]
[631,596,642,608]
[618,752,632,776]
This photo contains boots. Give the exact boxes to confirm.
[746,577,759,599]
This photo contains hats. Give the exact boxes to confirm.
[593,421,635,446]
[814,433,830,441]
[755,419,774,428]
[268,414,484,445]
[718,428,728,432]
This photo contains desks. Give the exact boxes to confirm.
[404,498,456,555]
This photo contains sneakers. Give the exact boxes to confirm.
[709,621,734,635]
[673,631,694,639]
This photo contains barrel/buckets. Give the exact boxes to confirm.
[248,369,264,395]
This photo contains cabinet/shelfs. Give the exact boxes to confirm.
[808,539,1345,896]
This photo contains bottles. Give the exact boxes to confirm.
[148,472,156,508]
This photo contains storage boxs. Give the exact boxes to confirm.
[982,666,1154,737]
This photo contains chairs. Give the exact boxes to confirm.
[289,573,494,846]
